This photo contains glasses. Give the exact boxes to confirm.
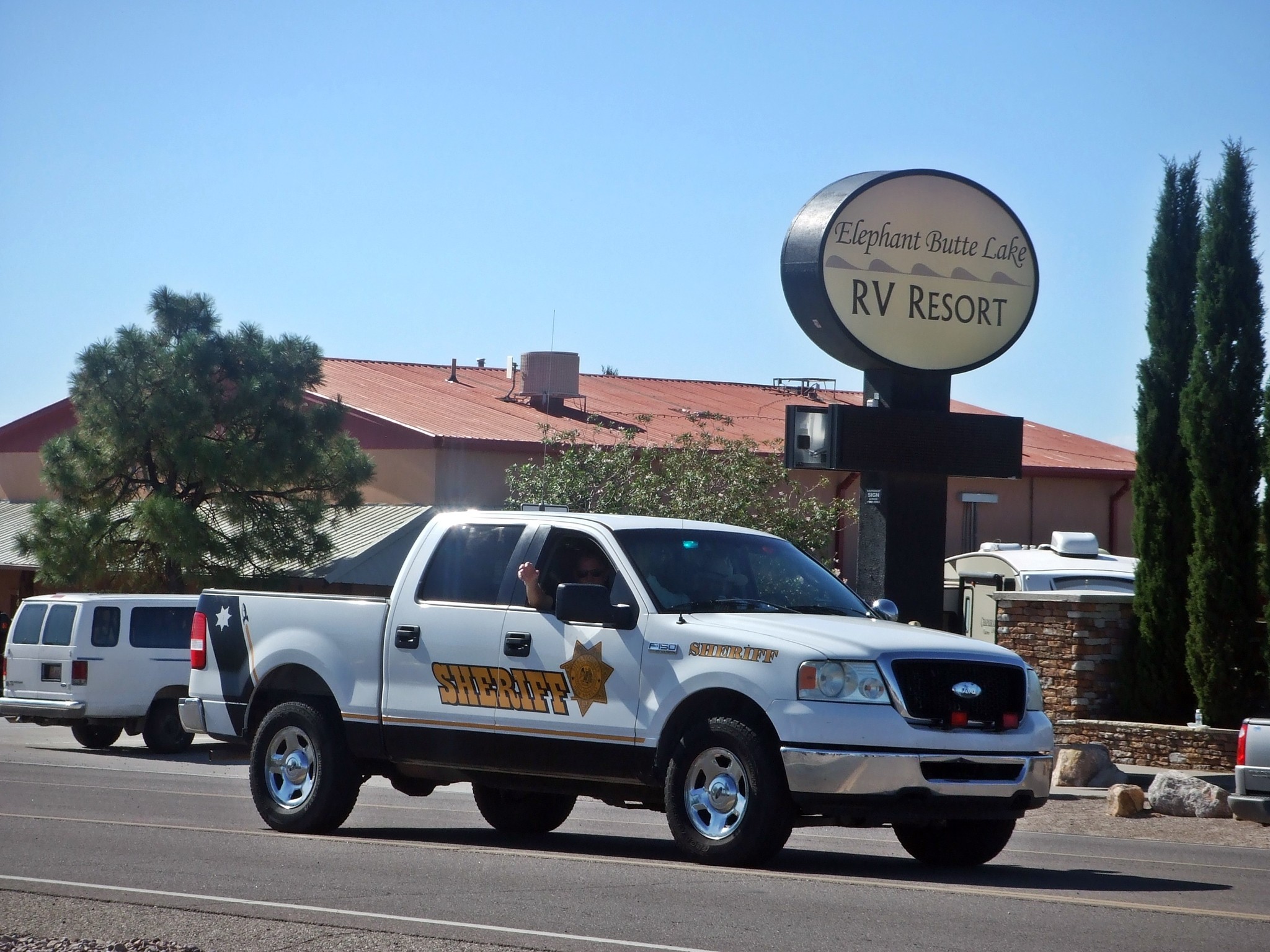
[576,568,606,578]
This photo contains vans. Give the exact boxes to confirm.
[0,592,201,758]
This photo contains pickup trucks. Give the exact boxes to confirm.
[177,508,1057,873]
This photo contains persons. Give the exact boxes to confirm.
[689,531,757,613]
[517,549,609,612]
